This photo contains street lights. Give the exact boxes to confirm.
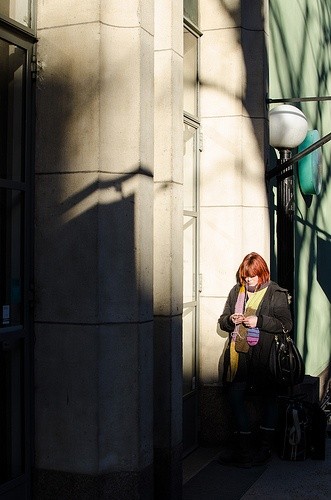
[268,102,307,340]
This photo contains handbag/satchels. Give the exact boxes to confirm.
[302,399,327,460]
[283,400,312,461]
[269,319,305,388]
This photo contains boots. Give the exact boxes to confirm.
[233,432,252,469]
[256,425,276,457]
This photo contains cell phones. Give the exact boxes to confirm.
[234,314,245,320]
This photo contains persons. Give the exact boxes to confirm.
[218,252,293,467]
